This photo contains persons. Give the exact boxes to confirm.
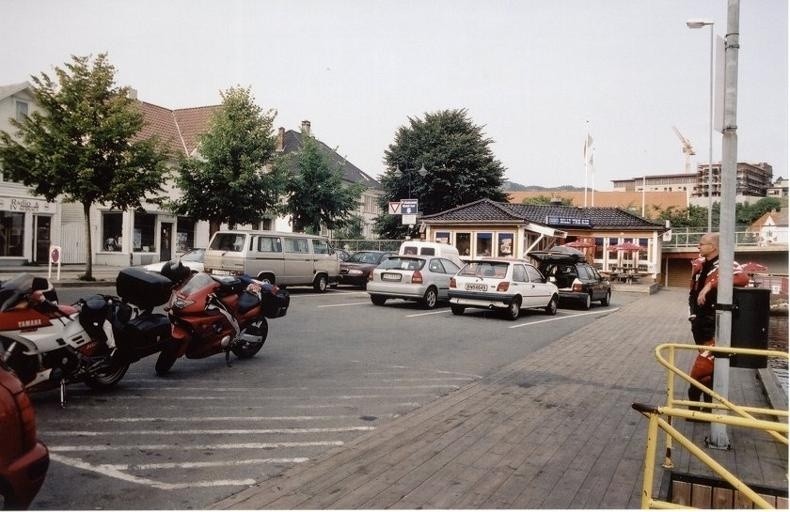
[684,230,750,423]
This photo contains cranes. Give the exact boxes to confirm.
[671,125,696,173]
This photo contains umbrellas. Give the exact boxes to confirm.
[604,240,648,282]
[560,240,598,253]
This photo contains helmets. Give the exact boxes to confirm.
[161,259,190,291]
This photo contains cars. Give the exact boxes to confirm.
[1,343,53,510]
[336,240,613,321]
[179,247,206,277]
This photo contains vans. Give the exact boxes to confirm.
[203,229,343,294]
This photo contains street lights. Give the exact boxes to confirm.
[687,21,714,234]
[394,158,428,200]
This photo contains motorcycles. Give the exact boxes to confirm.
[154,258,290,380]
[0,260,173,407]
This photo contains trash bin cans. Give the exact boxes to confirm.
[730,288,770,368]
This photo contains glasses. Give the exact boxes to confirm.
[699,243,713,246]
